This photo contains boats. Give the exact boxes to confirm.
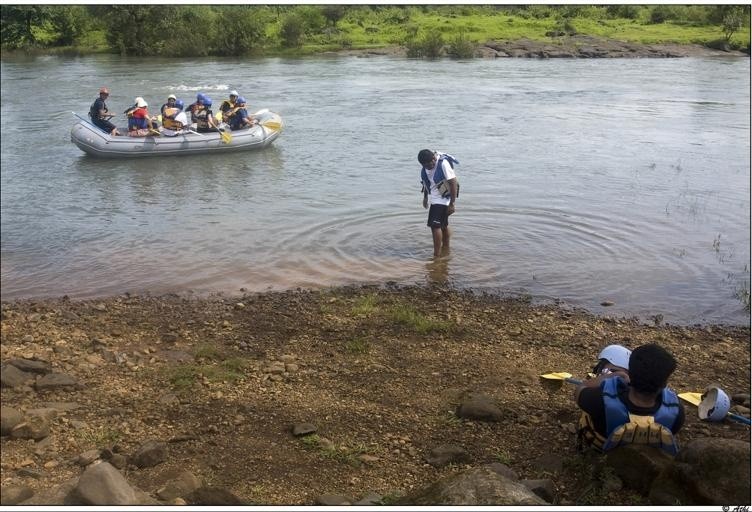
[66,109,282,157]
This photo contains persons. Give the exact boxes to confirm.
[89,90,120,137]
[124,91,253,132]
[416,149,458,255]
[573,341,685,452]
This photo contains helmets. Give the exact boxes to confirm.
[229,90,239,100]
[237,97,246,104]
[597,343,633,372]
[99,87,108,94]
[135,96,148,108]
[168,94,176,101]
[196,92,212,107]
[175,98,184,106]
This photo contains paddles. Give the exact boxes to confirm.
[255,123,281,130]
[216,127,232,144]
[540,372,583,385]
[677,392,751,425]
